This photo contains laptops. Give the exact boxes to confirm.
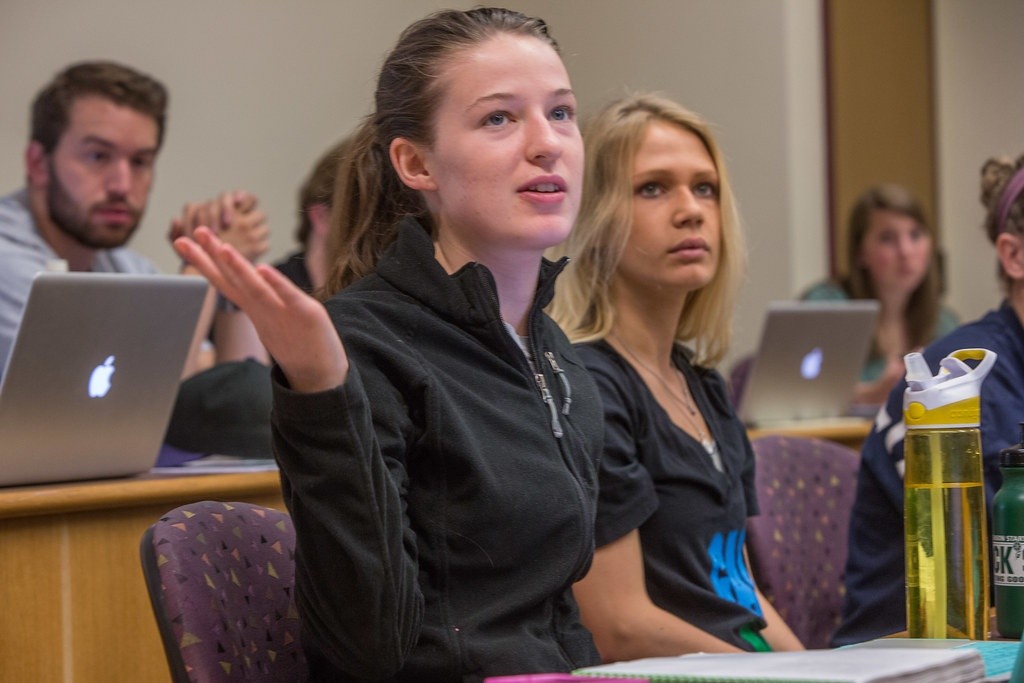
[0,270,210,487]
[737,299,880,427]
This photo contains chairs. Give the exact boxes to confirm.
[140,499,310,683]
[736,434,863,647]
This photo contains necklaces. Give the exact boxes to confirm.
[612,332,696,415]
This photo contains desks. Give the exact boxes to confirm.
[0,415,877,683]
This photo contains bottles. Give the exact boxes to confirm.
[901,348,996,639]
[993,422,1024,640]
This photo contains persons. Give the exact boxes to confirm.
[0,60,272,380]
[542,92,806,664]
[832,154,1024,645]
[173,4,603,683]
[270,139,354,296]
[801,182,959,418]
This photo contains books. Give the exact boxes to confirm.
[572,647,985,683]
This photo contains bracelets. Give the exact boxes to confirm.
[218,296,240,311]
